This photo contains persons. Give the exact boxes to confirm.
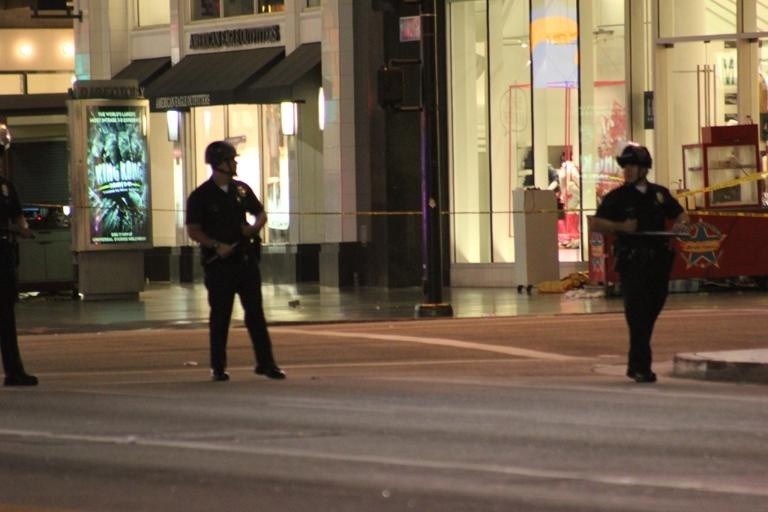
[1,122,39,386]
[186,141,285,381]
[525,151,560,190]
[592,142,690,386]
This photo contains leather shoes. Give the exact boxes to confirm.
[213,368,229,380]
[4,374,38,386]
[629,367,653,382]
[627,371,656,381]
[255,360,285,379]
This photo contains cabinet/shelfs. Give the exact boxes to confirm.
[16,229,77,283]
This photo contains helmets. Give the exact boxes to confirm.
[616,145,651,168]
[206,141,239,162]
